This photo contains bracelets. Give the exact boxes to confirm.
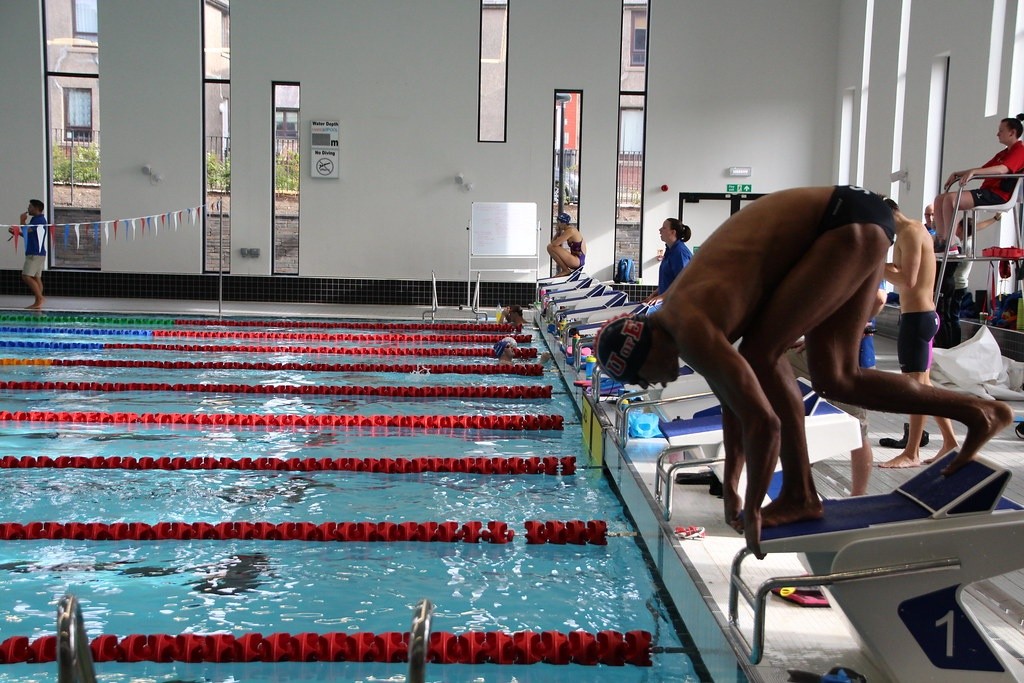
[993,216,1001,221]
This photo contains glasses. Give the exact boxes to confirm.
[505,345,510,348]
[924,213,935,217]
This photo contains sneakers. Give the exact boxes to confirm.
[934,237,959,257]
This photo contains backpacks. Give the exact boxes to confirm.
[618,256,637,284]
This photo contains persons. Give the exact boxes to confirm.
[934,112,1024,257]
[9,200,47,309]
[923,203,1002,349]
[499,305,527,323]
[493,341,550,362]
[640,218,694,315]
[593,185,1012,561]
[788,288,887,495]
[547,213,586,277]
[878,196,959,468]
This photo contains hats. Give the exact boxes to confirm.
[501,337,517,347]
[557,213,571,223]
[493,340,509,357]
[593,313,653,384]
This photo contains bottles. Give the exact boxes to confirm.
[585,355,597,380]
[496,303,502,323]
[620,399,630,412]
[549,304,556,320]
[558,319,567,342]
[544,296,552,315]
[541,289,547,313]
[572,333,581,356]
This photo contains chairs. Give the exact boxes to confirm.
[537,264,1009,552]
[961,177,1023,258]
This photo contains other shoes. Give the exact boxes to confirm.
[1015,421,1024,439]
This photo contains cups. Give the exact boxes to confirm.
[979,312,988,324]
[636,278,642,284]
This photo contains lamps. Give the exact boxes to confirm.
[730,167,751,177]
[888,168,911,190]
